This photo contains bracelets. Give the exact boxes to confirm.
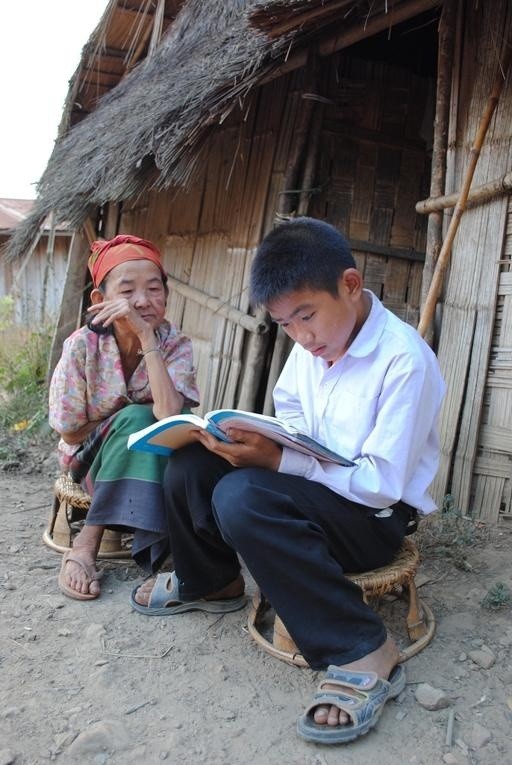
[137,347,161,356]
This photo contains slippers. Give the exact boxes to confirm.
[56,548,107,602]
[128,568,249,617]
[296,661,408,748]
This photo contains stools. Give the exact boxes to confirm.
[42,472,136,558]
[246,536,436,670]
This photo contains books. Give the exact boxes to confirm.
[127,407,358,468]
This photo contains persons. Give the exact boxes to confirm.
[49,234,202,601]
[125,216,447,747]
